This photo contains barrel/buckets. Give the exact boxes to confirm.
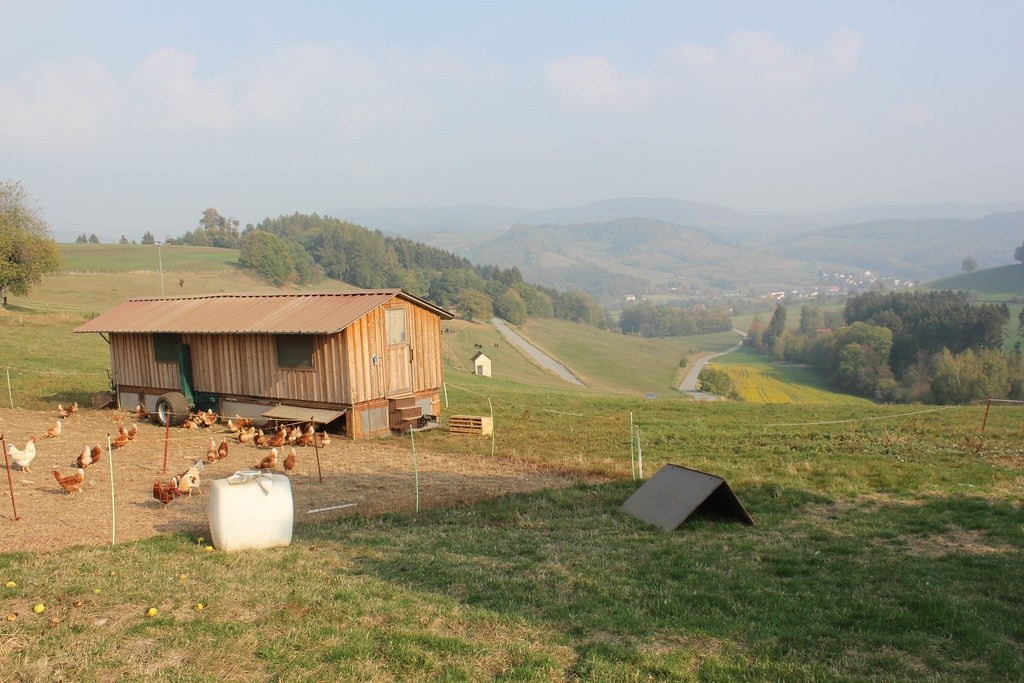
[208,474,294,553]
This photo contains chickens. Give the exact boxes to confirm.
[6,400,332,513]
